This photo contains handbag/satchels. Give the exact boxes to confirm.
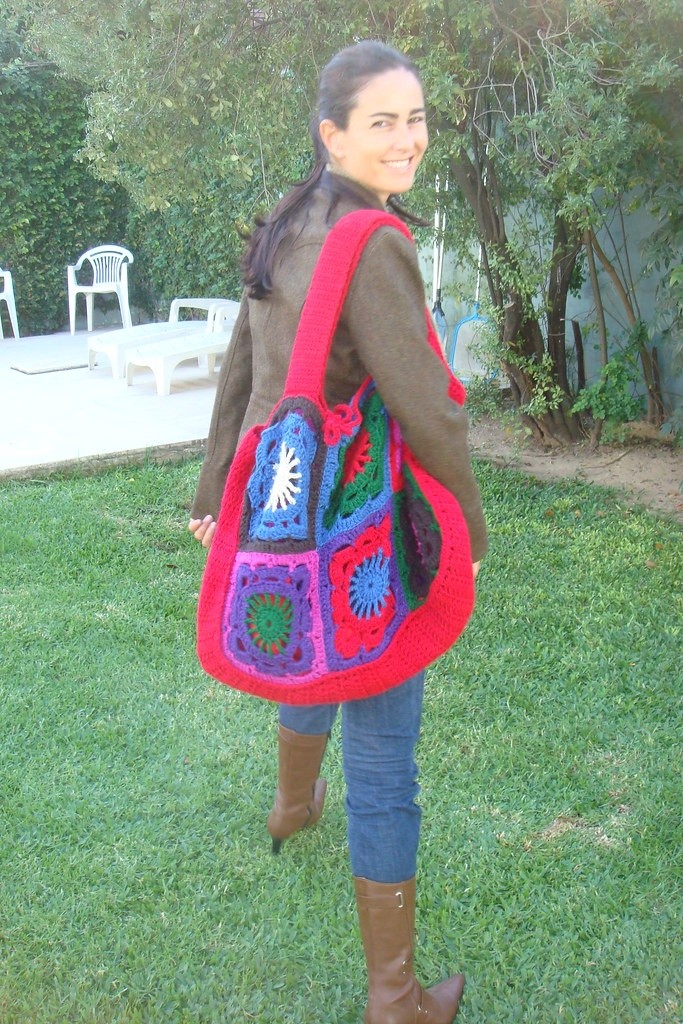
[197,208,474,705]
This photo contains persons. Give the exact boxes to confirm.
[188,41,489,1024]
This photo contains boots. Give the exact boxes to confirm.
[266,720,327,854]
[351,874,463,1024]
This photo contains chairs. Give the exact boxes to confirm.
[87,299,241,396]
[68,245,135,335]
[0,267,20,339]
